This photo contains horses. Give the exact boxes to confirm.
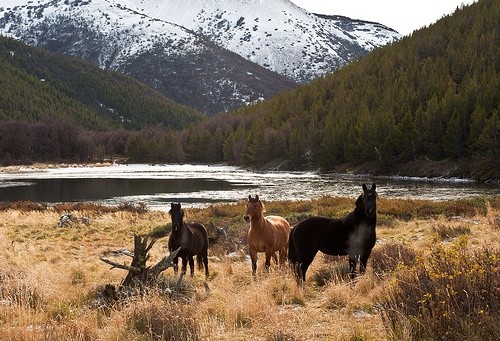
[288,183,378,282]
[243,194,290,276]
[168,202,209,277]
